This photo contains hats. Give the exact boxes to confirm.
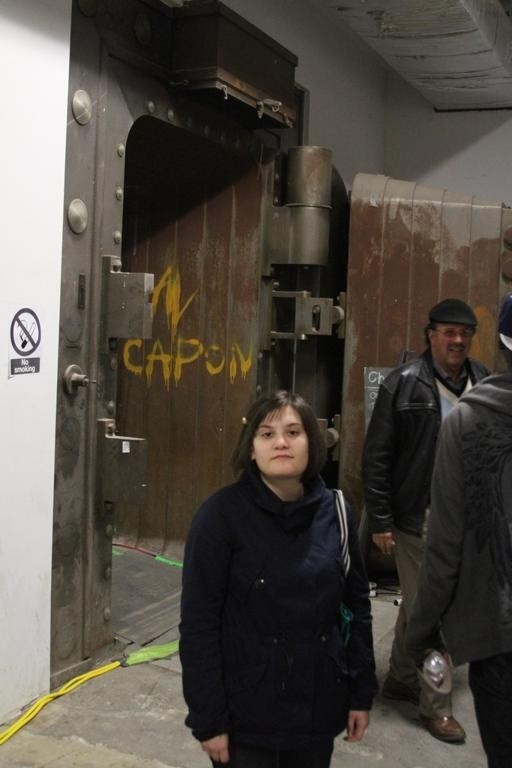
[428,299,478,326]
[498,295,512,351]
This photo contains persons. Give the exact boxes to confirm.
[179,391,379,768]
[403,289,512,767]
[362,297,494,742]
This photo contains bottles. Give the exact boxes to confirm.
[415,646,453,695]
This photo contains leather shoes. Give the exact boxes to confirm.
[424,716,465,742]
[384,681,418,705]
